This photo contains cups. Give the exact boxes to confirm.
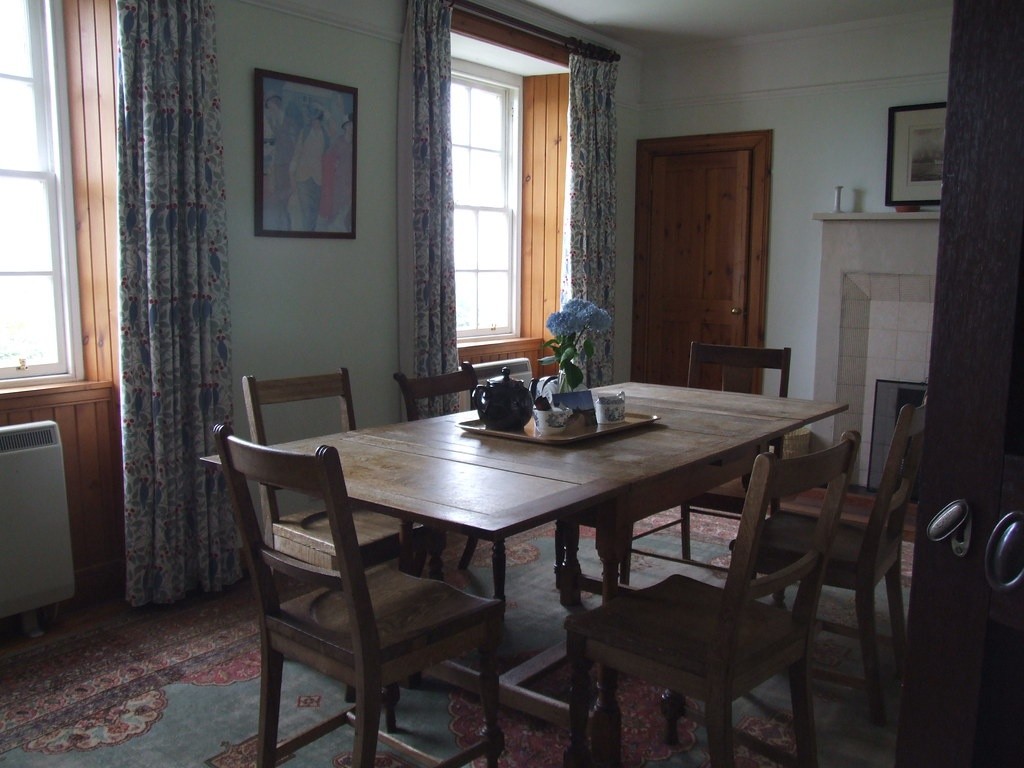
[532,409,568,436]
[536,376,558,404]
[596,391,625,425]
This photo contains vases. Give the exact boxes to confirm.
[557,369,569,392]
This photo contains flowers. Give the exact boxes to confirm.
[537,298,612,389]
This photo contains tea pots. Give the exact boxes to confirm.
[472,367,539,430]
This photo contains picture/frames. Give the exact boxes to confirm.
[885,101,947,206]
[254,67,358,239]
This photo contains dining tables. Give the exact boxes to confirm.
[199,381,850,767]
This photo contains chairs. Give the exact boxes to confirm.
[565,430,862,767]
[392,360,566,589]
[242,367,447,701]
[728,387,929,722]
[215,422,506,767]
[620,340,791,584]
